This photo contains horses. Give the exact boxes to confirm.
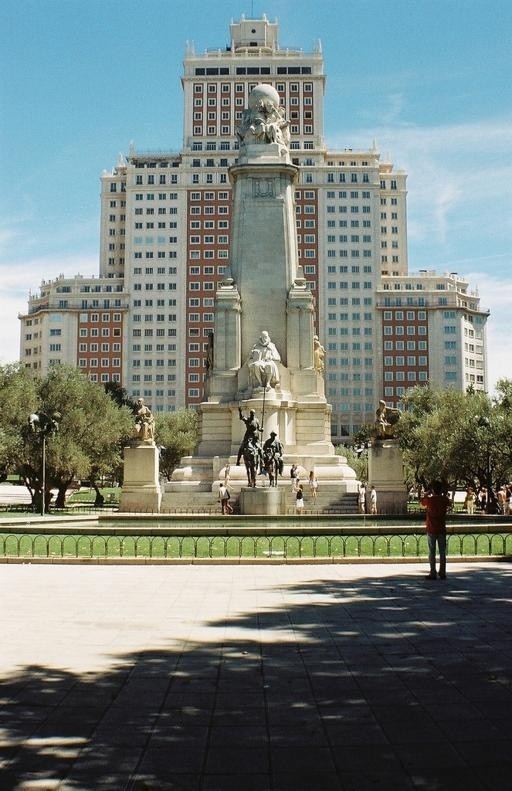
[242,435,259,488]
[264,444,280,487]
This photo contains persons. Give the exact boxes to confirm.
[376,400,400,435]
[359,484,366,514]
[421,480,452,580]
[466,481,512,515]
[258,432,284,477]
[219,464,234,515]
[291,464,304,515]
[371,486,377,514]
[236,406,264,466]
[309,471,317,497]
[250,331,281,388]
[135,398,155,442]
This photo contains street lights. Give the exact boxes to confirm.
[27,412,62,516]
